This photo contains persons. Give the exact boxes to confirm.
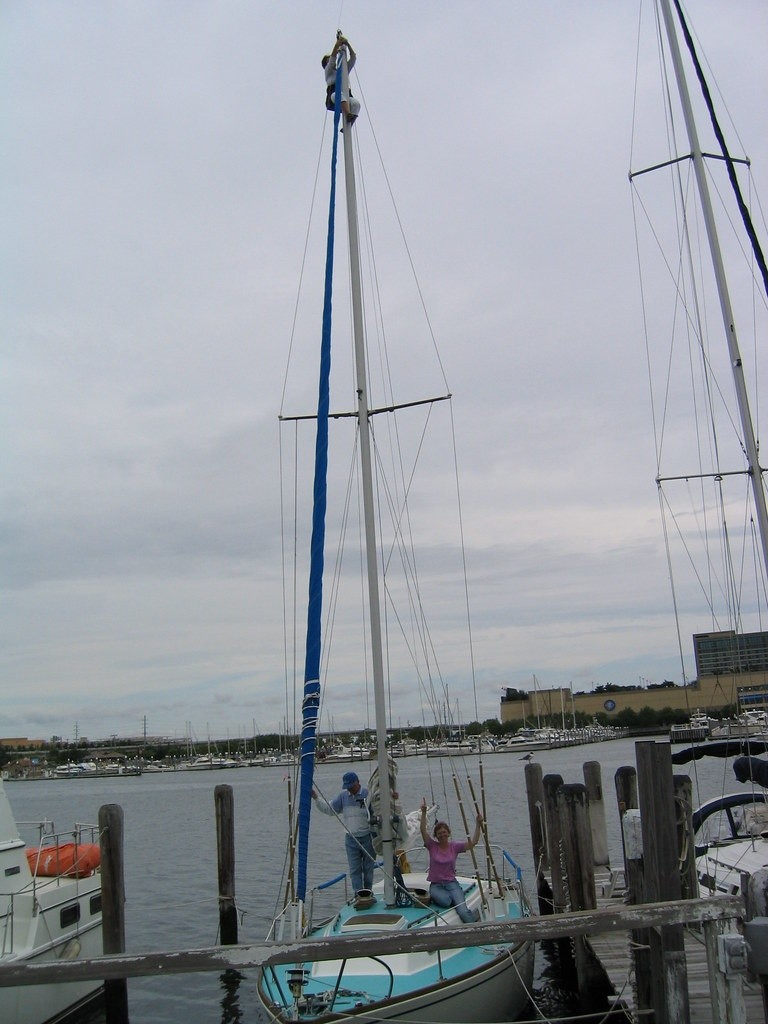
[311,771,377,904]
[420,797,484,923]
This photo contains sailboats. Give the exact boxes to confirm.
[257,30,536,1023]
[187,710,371,770]
[388,674,630,757]
[627,0,768,901]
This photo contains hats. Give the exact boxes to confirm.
[342,772,358,789]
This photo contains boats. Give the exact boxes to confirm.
[0,781,107,1024]
[52,761,140,776]
[670,708,768,743]
[142,761,193,773]
